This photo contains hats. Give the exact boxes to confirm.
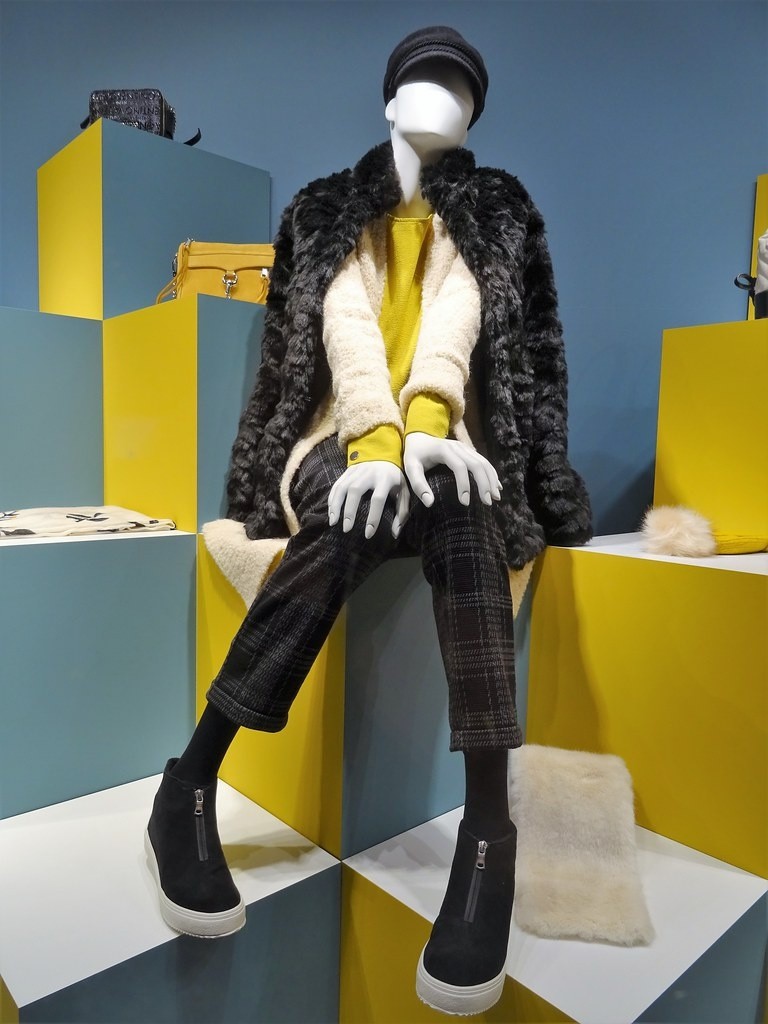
[383,26,488,130]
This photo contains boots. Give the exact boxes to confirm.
[142,756,248,940]
[414,819,517,1015]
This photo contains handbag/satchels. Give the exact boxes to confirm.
[155,238,276,304]
[80,90,203,146]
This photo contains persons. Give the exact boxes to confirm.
[144,24,586,1014]
